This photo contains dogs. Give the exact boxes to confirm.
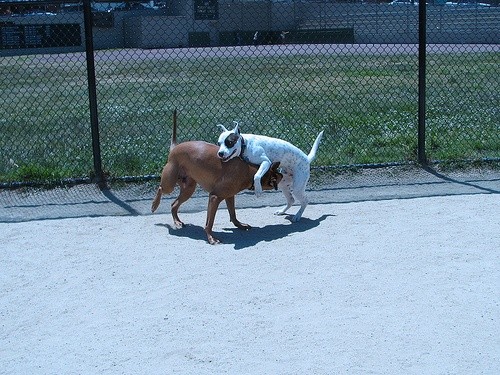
[151,106,284,245]
[216,121,325,222]
